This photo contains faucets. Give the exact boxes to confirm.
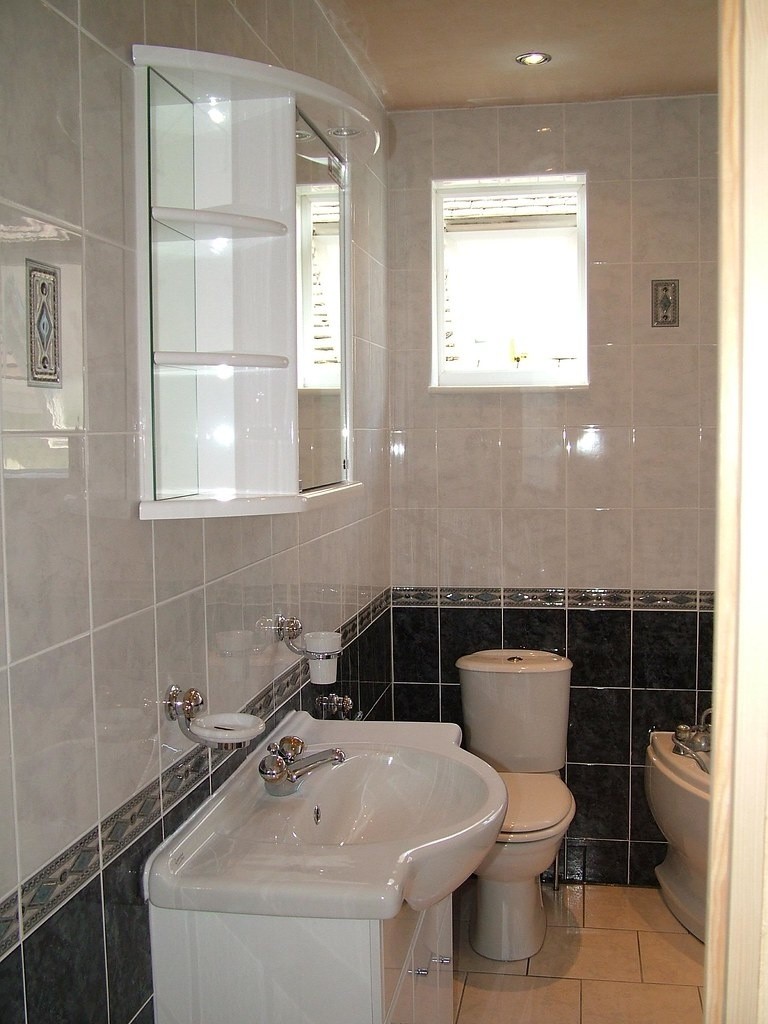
[256,735,346,796]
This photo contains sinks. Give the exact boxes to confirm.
[137,709,508,909]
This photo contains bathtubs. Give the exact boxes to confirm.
[644,733,711,948]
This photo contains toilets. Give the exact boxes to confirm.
[453,647,576,962]
